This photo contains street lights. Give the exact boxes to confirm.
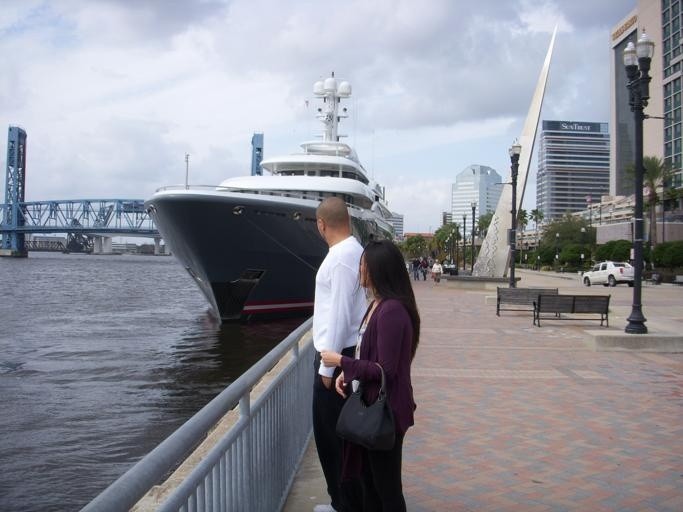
[621,24,655,336]
[535,237,541,270]
[628,216,634,266]
[551,232,560,273]
[578,227,585,275]
[522,240,528,269]
[469,197,477,276]
[507,135,522,288]
[443,211,465,272]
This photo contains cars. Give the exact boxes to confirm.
[580,259,634,287]
[441,258,457,272]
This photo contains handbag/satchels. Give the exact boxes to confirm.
[333,360,397,454]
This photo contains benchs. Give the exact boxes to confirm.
[646,274,660,285]
[533,293,611,328]
[673,274,683,287]
[496,286,560,318]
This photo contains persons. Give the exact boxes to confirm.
[312,196,377,512]
[319,240,421,512]
[412,254,449,288]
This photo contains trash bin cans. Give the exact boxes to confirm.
[160,245,165,253]
[651,272,661,284]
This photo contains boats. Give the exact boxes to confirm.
[142,71,397,324]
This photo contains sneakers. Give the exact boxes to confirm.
[312,503,335,512]
